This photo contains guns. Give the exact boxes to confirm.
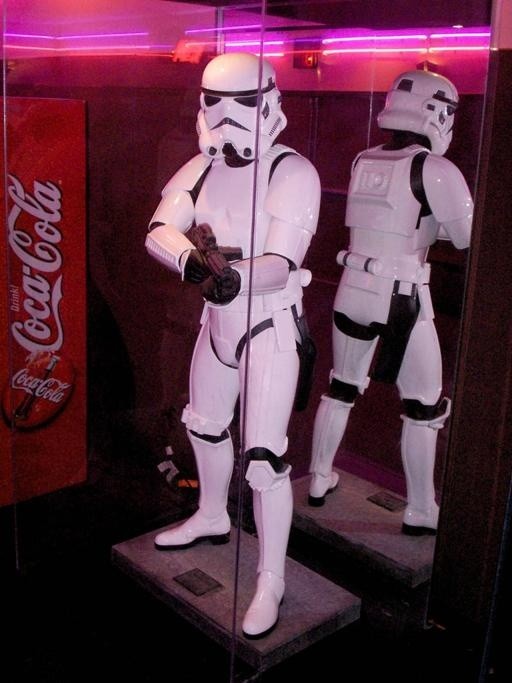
[188,222,243,299]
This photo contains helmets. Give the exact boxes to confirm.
[195,53,288,161]
[377,71,461,157]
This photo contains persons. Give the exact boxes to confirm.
[305,68,475,537]
[145,51,324,642]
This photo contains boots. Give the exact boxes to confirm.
[241,459,294,639]
[155,429,234,549]
[401,398,452,537]
[306,394,356,506]
